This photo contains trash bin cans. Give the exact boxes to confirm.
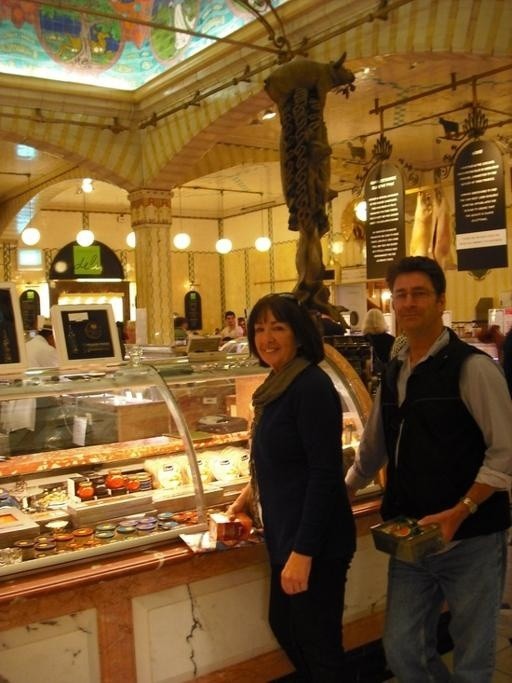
[461,495,478,514]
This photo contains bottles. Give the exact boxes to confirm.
[39,319,52,331]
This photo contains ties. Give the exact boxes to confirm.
[16,247,44,271]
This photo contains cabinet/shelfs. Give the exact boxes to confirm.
[107,469,124,487]
[14,509,232,561]
[135,472,153,489]
[94,480,106,494]
[126,475,140,490]
[76,482,93,497]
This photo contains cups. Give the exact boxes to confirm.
[265,293,301,306]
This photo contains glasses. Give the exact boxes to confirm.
[262,105,279,121]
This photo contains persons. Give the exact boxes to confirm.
[220,290,356,683]
[174,318,190,338]
[360,307,396,377]
[484,323,503,357]
[114,321,128,359]
[5,323,58,456]
[344,254,512,681]
[237,317,246,337]
[220,310,243,339]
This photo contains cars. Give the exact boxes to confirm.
[129,346,142,365]
